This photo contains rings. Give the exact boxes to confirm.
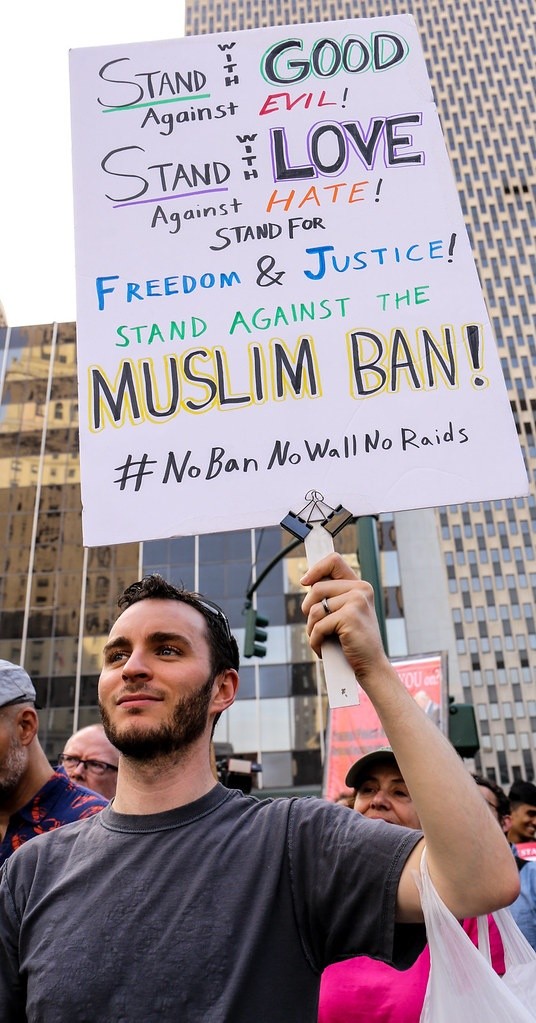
[322,598,332,615]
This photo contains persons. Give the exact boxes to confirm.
[504,777,536,845]
[0,659,110,868]
[320,747,506,1023]
[57,724,119,801]
[414,692,439,726]
[0,552,520,1023]
[470,772,536,951]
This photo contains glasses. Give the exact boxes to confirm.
[57,752,119,777]
[196,596,231,641]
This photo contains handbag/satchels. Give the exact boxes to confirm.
[413,843,536,1023]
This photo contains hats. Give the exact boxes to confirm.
[0,659,36,706]
[348,745,397,787]
